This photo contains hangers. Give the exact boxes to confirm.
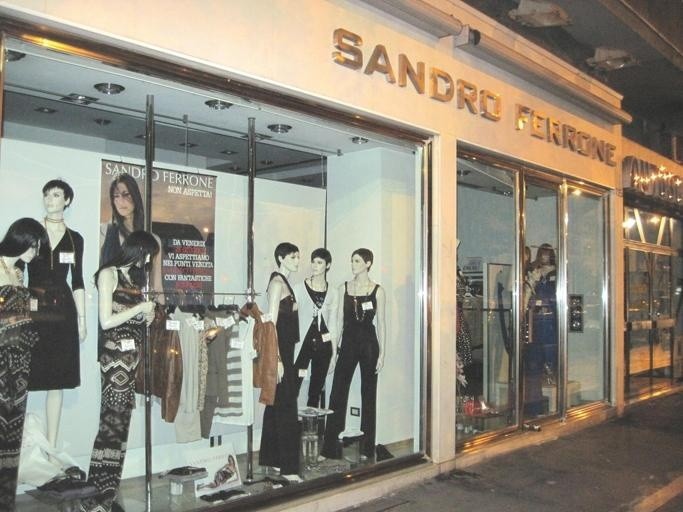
[148,289,258,328]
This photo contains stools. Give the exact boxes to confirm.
[338,430,365,472]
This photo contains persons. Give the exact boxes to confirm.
[197,455,238,491]
[259,242,302,483]
[0,217,50,512]
[316,247,385,462]
[457,243,557,433]
[293,248,340,435]
[79,231,159,512]
[15,179,87,449]
[98,172,164,308]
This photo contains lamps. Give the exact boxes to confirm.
[509,0,568,29]
[586,45,638,74]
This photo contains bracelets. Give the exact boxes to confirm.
[277,355,282,362]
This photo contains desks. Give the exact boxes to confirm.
[296,407,334,472]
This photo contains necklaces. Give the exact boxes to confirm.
[1,259,24,290]
[43,217,75,269]
[353,279,372,321]
[310,276,326,290]
